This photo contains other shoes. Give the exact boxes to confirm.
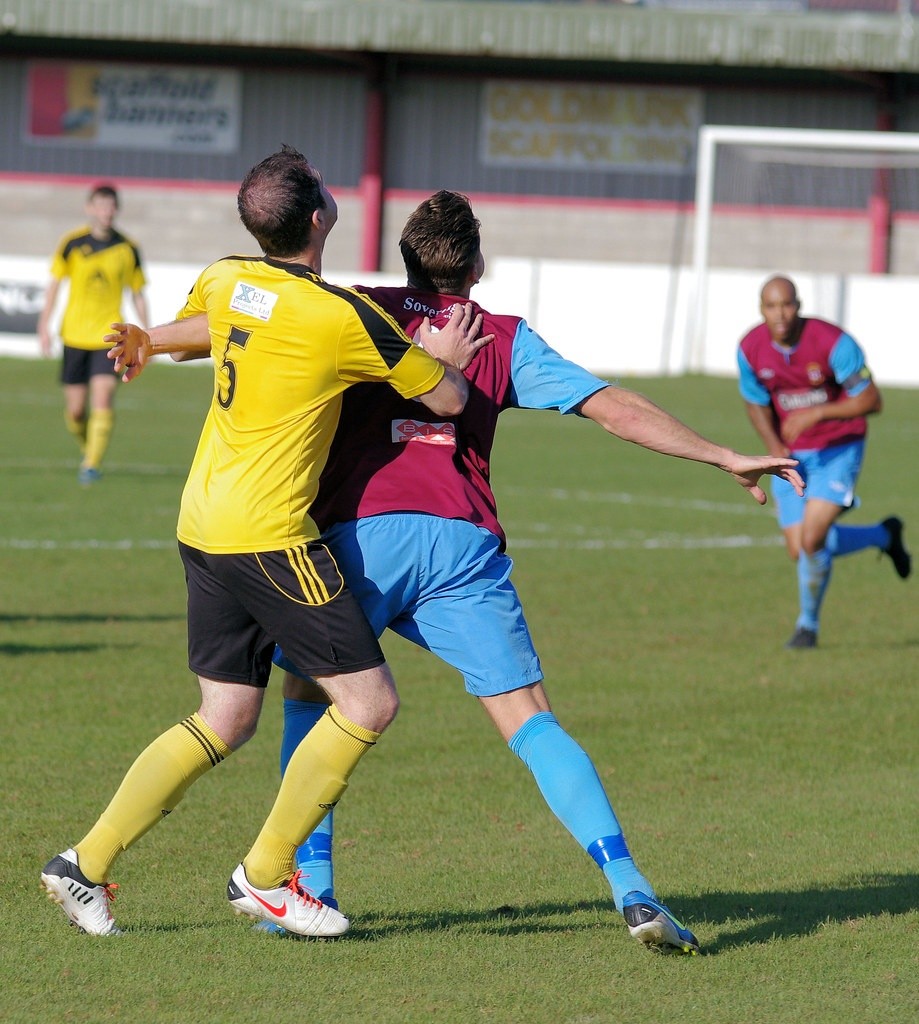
[80,470,98,482]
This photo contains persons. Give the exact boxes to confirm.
[38,147,498,938]
[39,184,150,484]
[106,185,804,957]
[736,277,914,651]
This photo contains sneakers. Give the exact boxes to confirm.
[38,848,124,935]
[225,862,349,935]
[623,891,699,956]
[786,626,815,647]
[880,518,910,578]
[250,897,339,940]
